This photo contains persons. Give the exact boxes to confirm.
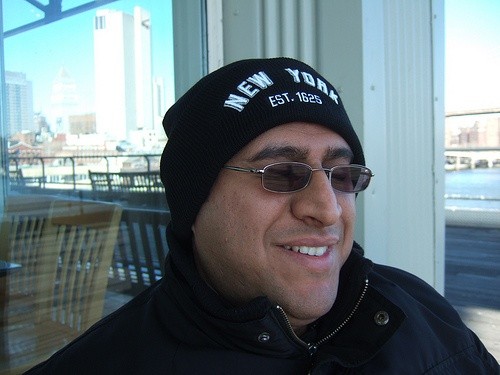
[21,57,500,375]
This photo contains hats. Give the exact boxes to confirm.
[159,57,366,245]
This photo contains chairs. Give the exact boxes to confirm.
[0,196,123,375]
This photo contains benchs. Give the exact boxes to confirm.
[88,170,165,203]
[9,168,47,194]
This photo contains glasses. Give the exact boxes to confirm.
[223,164,374,193]
